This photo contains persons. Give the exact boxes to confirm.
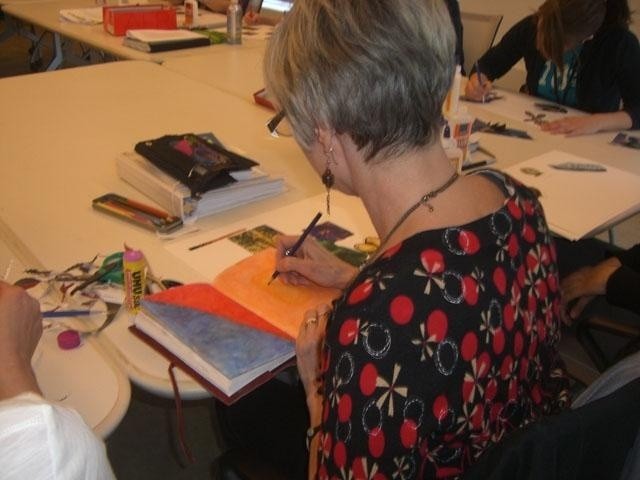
[463,0,640,139]
[554,239,640,326]
[1,281,118,479]
[261,0,571,479]
[182,0,249,19]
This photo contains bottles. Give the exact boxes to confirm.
[441,66,461,113]
[443,126,462,176]
[183,0,199,29]
[227,1,243,43]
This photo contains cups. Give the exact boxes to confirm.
[444,107,473,164]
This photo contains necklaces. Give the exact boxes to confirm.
[374,169,461,254]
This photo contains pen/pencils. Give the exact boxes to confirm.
[39,311,115,317]
[96,194,178,231]
[250,3,253,18]
[475,60,485,104]
[267,213,322,286]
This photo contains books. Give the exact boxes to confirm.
[503,148,640,241]
[124,38,210,53]
[117,141,283,224]
[128,245,344,407]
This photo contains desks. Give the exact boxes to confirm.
[4,63,383,398]
[4,1,281,65]
[162,31,640,243]
[1,243,126,446]
[467,243,639,480]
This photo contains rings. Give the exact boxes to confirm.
[318,310,333,316]
[305,317,317,325]
